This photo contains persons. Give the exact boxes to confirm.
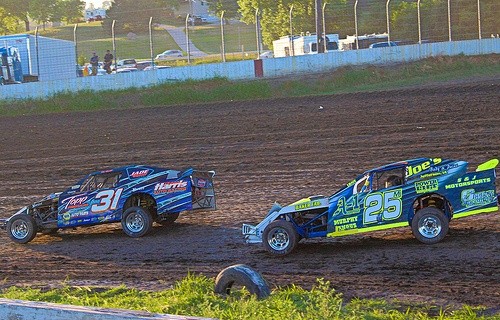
[78,53,84,66]
[104,49,113,74]
[319,32,330,53]
[89,51,99,76]
[385,175,402,190]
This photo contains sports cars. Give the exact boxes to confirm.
[240,157,500,257]
[0,165,216,244]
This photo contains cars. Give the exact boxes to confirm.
[155,50,184,59]
[76,59,172,79]
